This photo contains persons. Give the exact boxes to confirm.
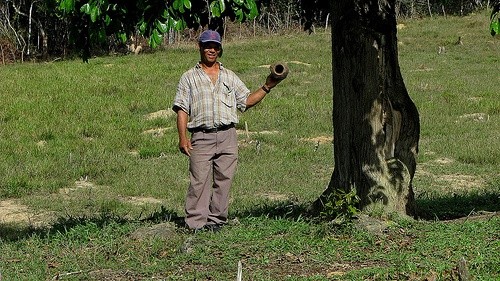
[168,31,279,233]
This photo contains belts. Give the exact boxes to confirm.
[195,122,235,134]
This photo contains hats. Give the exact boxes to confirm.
[198,30,223,47]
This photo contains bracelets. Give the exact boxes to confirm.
[262,86,270,93]
